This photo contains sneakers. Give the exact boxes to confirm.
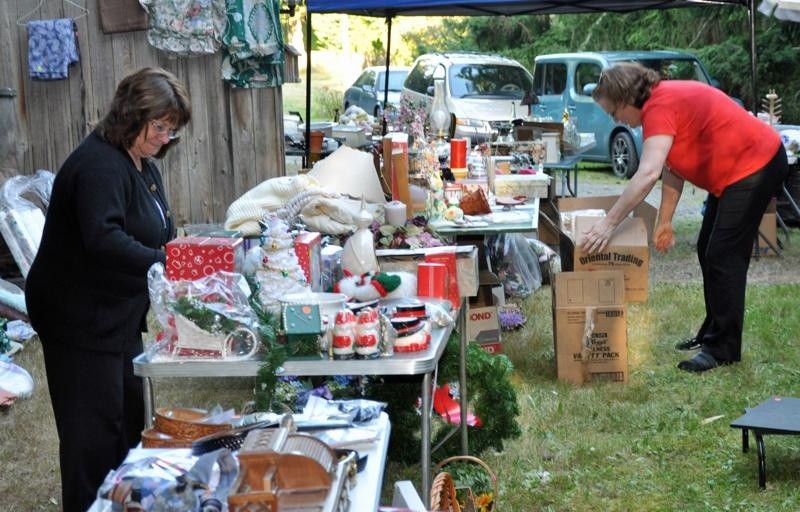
[677,352,733,374]
[674,336,702,353]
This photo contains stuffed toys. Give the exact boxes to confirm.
[324,269,435,359]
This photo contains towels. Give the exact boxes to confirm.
[27,20,80,79]
[98,1,148,35]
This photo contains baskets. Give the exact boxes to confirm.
[430,454,499,512]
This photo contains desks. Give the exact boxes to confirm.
[128,274,473,510]
[86,399,433,512]
[277,132,597,306]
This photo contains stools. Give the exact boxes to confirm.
[729,395,800,489]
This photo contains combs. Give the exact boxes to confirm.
[193,419,281,460]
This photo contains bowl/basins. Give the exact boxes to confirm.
[299,122,333,138]
[278,292,347,329]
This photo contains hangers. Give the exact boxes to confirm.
[16,0,90,26]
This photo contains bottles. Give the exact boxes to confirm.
[332,307,380,360]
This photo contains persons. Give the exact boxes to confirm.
[23,63,194,512]
[577,59,791,373]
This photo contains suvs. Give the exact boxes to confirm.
[400,53,534,149]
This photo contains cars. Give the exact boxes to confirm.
[527,50,721,179]
[343,66,412,132]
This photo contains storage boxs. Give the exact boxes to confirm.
[749,198,778,257]
[556,196,658,304]
[463,270,504,356]
[548,273,629,387]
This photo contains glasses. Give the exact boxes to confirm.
[152,119,181,140]
